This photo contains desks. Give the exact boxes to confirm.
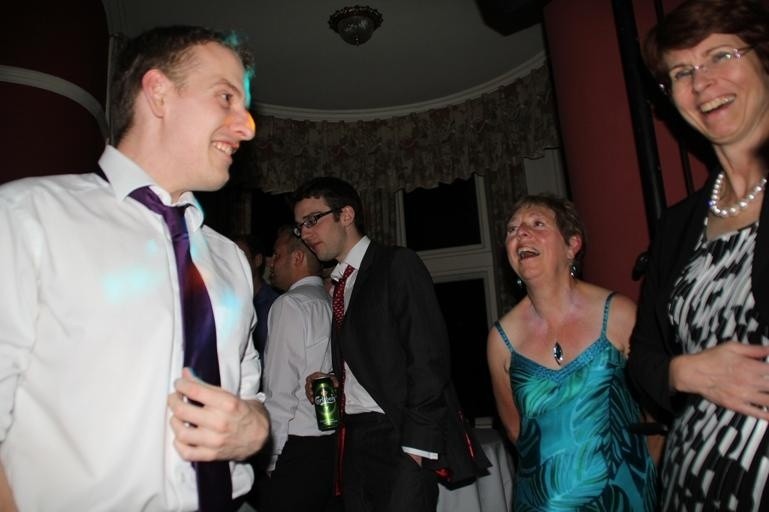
[436,425,513,512]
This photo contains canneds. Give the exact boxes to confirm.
[311,375,341,431]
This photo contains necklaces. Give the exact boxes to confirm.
[528,292,571,366]
[705,171,766,217]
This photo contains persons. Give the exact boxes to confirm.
[623,2,768,512]
[293,176,493,511]
[485,193,662,511]
[0,26,279,512]
[224,222,346,510]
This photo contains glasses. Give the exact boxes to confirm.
[291,207,341,238]
[652,43,755,97]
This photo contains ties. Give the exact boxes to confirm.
[93,160,232,512]
[329,264,356,422]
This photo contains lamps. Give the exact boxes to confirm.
[326,5,385,47]
